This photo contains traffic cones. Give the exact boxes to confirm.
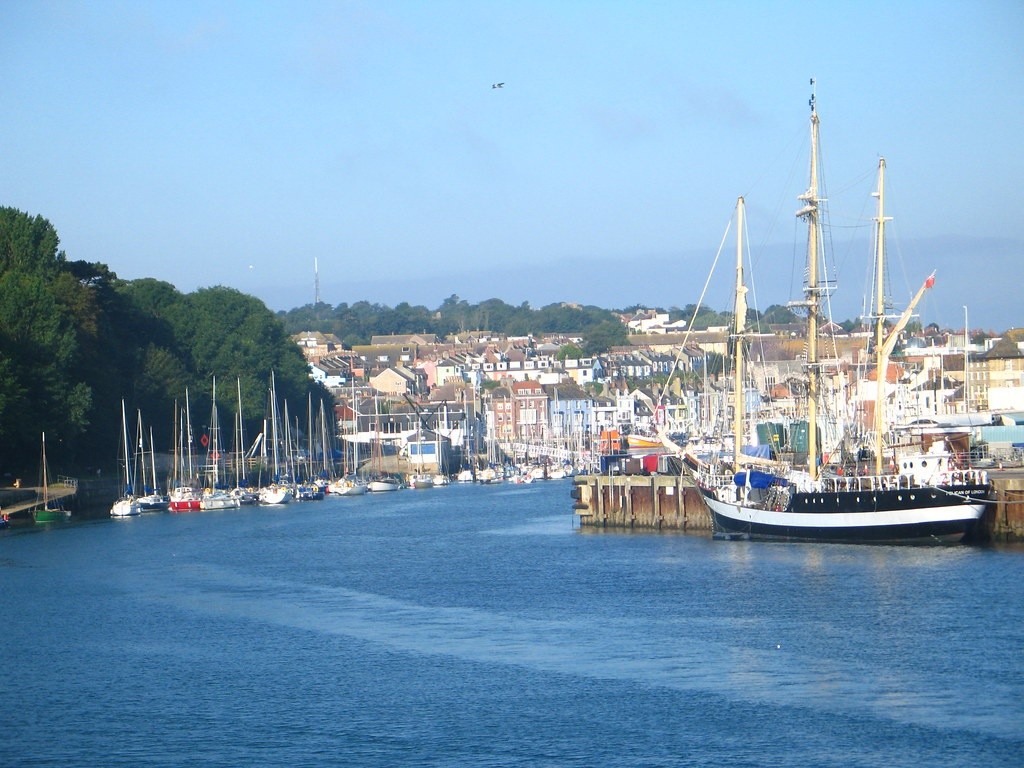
[889,457,894,469]
[998,461,1006,472]
[864,464,869,476]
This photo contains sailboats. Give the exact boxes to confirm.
[651,75,994,547]
[33,431,72,524]
[108,369,596,515]
